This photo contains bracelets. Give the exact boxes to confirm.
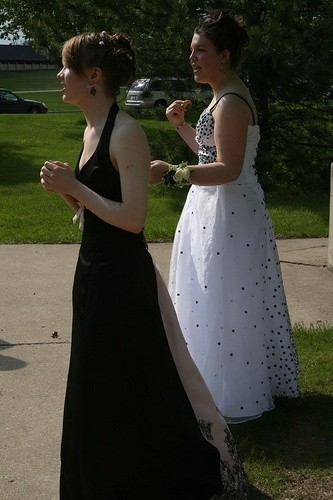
[161,162,195,188]
[176,121,188,131]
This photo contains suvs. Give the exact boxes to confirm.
[123,76,212,116]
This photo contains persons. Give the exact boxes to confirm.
[40,32,276,500]
[148,11,306,423]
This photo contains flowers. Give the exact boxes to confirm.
[162,161,194,189]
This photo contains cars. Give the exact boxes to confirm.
[0,88,48,114]
